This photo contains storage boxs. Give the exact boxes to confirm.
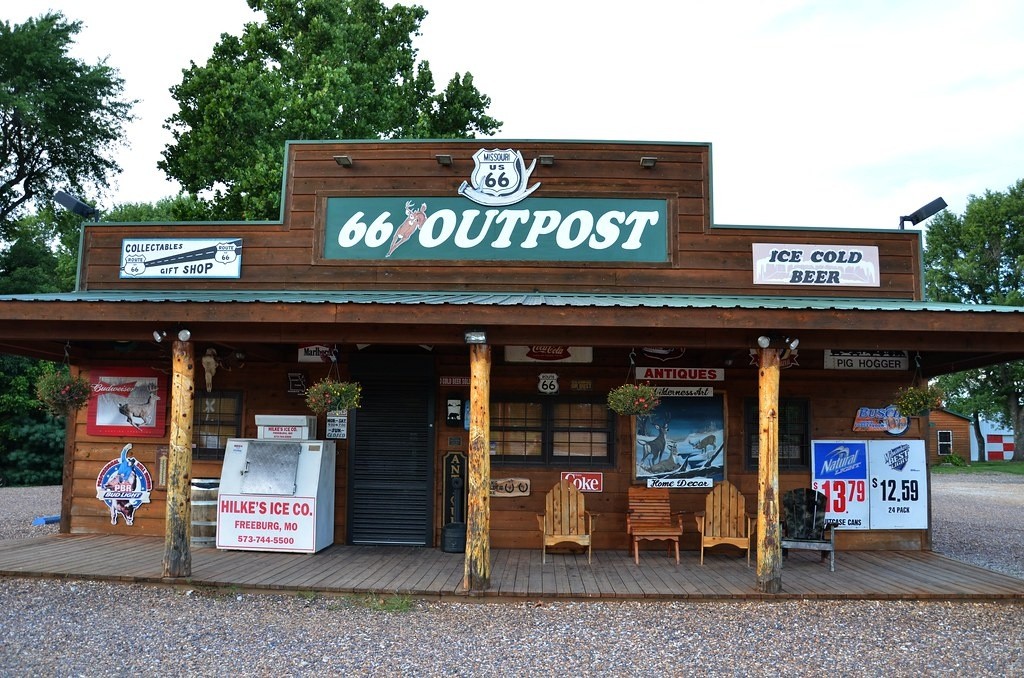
[254,414,316,440]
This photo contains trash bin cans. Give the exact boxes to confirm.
[216,436,336,552]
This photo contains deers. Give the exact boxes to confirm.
[118,383,161,432]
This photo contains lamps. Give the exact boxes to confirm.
[152,327,169,343]
[757,334,775,348]
[333,155,354,168]
[899,197,947,231]
[435,154,453,166]
[781,334,799,359]
[175,324,191,341]
[53,191,100,222]
[640,156,657,169]
[539,155,553,167]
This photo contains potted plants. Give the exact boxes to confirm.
[893,386,942,418]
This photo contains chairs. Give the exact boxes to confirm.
[694,480,757,568]
[537,480,600,565]
[625,487,689,565]
[778,488,839,573]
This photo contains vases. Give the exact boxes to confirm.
[327,403,343,412]
[632,408,641,415]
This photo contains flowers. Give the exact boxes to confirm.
[304,378,362,415]
[34,369,98,415]
[606,380,660,416]
